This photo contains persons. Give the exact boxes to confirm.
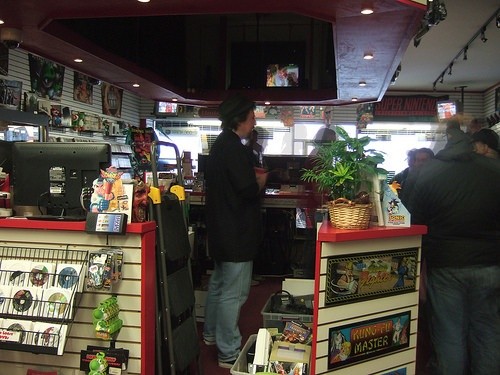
[308,127,337,157]
[204,93,269,331]
[389,112,500,375]
[244,126,269,155]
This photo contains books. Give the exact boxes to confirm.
[267,360,307,375]
[276,320,313,345]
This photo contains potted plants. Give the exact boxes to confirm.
[301,125,388,230]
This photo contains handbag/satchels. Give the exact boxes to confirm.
[269,287,314,315]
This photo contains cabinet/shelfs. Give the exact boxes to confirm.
[0,245,90,356]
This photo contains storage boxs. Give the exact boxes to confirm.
[230,334,258,375]
[261,293,313,333]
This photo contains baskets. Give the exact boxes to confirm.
[328,198,375,230]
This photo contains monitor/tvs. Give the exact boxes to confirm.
[154,102,178,117]
[10,142,111,221]
[264,65,299,87]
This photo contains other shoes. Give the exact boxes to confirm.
[202,336,217,345]
[219,360,236,369]
